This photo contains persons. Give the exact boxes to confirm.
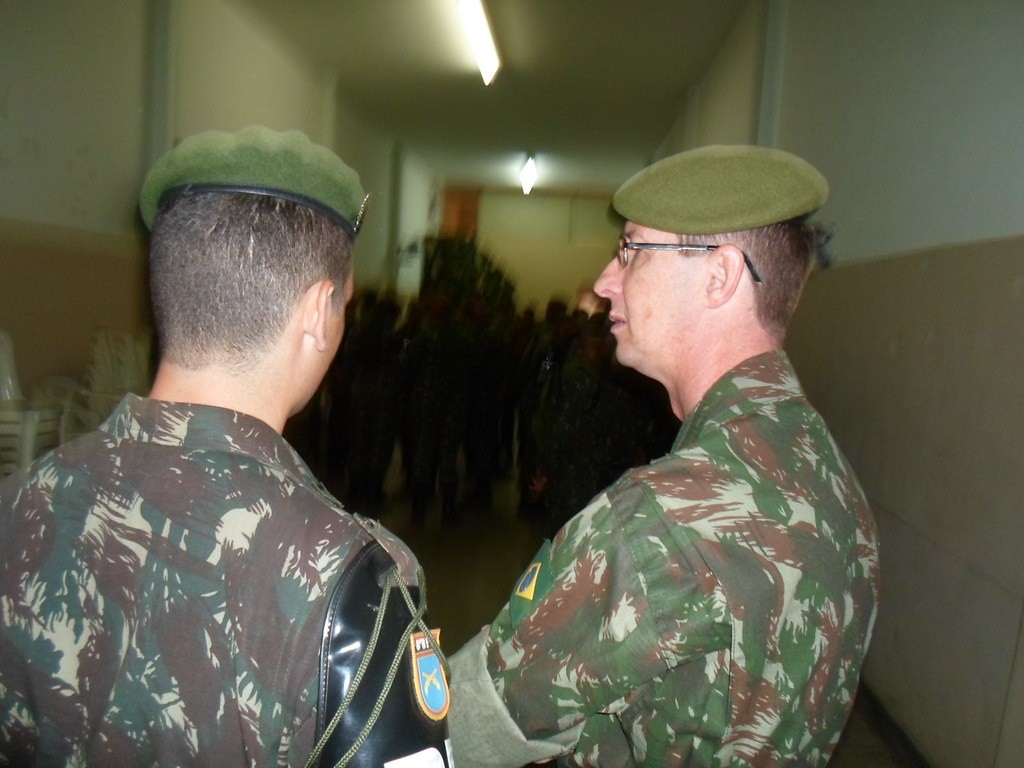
[445,146,880,768]
[281,294,681,538]
[0,126,456,768]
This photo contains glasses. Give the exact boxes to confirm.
[617,237,762,282]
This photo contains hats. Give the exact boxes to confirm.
[139,126,370,239]
[611,145,829,235]
